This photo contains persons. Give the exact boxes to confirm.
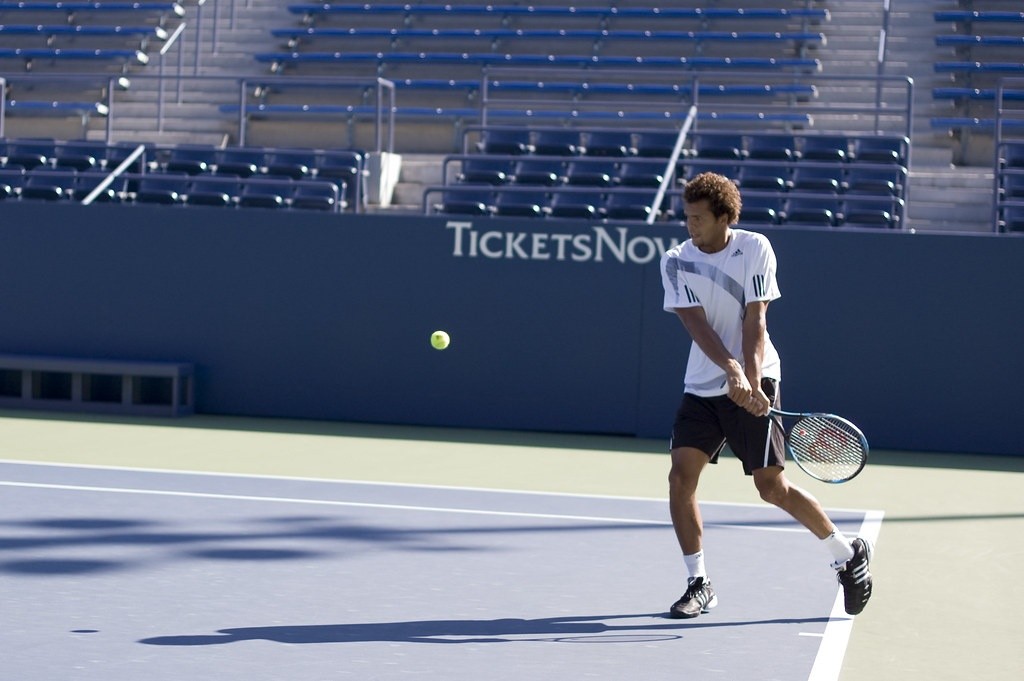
[660,171,873,618]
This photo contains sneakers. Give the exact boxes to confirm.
[829,538,872,615]
[670,576,719,619]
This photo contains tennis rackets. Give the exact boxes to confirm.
[720,380,870,485]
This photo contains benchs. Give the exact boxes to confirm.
[0,1,1024,127]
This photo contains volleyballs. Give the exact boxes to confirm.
[430,331,450,350]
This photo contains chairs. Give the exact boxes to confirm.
[0,130,1024,232]
